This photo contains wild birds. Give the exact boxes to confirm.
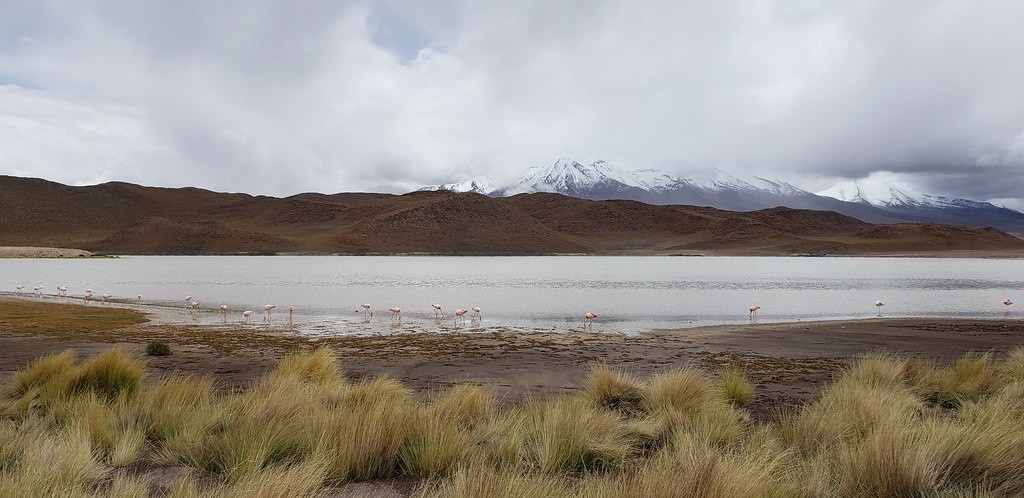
[135,294,144,306]
[14,284,26,297]
[430,303,445,320]
[219,304,229,324]
[747,304,761,322]
[102,293,113,305]
[263,303,297,328]
[83,289,96,305]
[453,309,468,325]
[471,306,483,322]
[582,311,598,327]
[873,300,887,316]
[32,286,45,300]
[55,287,69,298]
[184,296,201,312]
[351,302,405,325]
[1002,298,1015,314]
[241,310,253,325]
